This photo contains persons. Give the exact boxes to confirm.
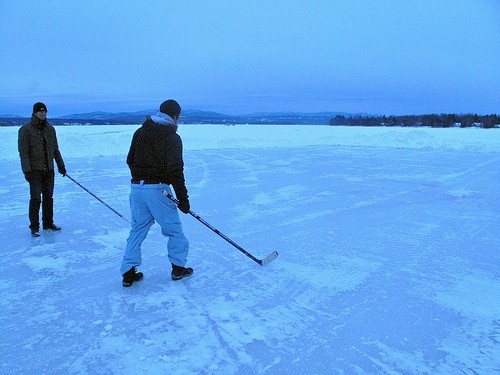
[119,98,194,287]
[17,99,67,237]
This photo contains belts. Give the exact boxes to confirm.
[130,179,170,184]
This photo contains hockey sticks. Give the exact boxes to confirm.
[64,173,131,225]
[161,189,279,266]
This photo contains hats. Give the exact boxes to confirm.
[33,101,47,113]
[160,99,181,114]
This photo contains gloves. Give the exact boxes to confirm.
[23,172,36,182]
[178,197,190,214]
[58,165,67,177]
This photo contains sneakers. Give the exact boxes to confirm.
[32,230,40,237]
[171,263,194,280]
[122,266,143,286]
[43,224,62,231]
